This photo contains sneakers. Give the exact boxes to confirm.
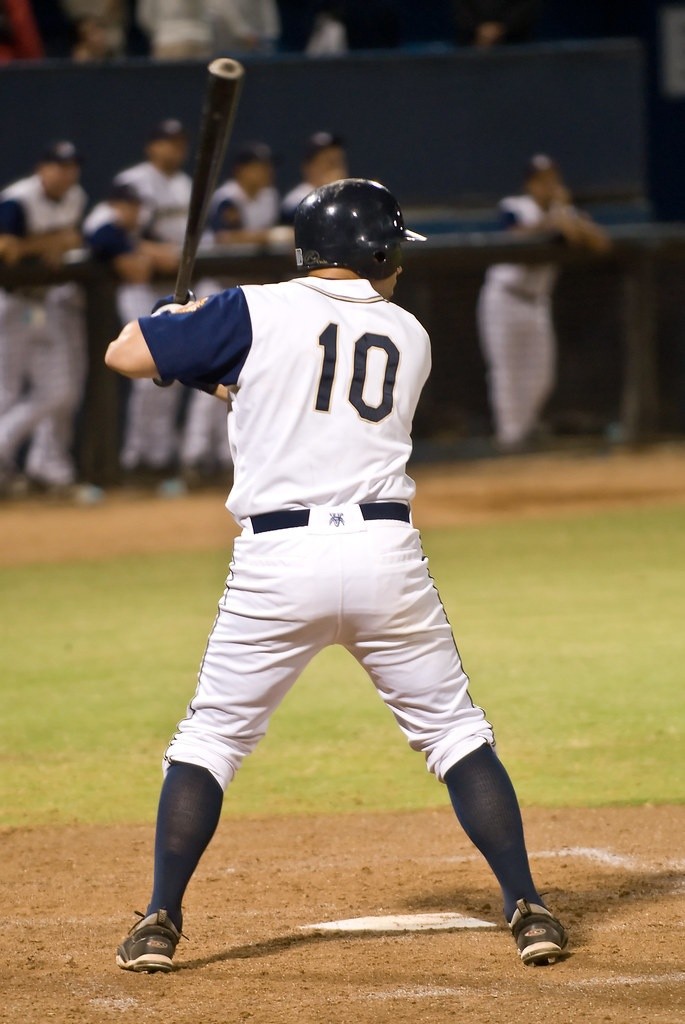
[115,911,190,973]
[507,896,568,966]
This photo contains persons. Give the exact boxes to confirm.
[476,153,611,459]
[105,176,574,973]
[0,0,358,498]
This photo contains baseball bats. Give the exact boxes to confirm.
[152,57,248,389]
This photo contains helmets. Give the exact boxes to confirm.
[44,139,81,162]
[293,177,429,277]
[524,155,559,174]
[147,120,190,144]
[108,183,142,206]
[299,131,346,168]
[237,145,276,164]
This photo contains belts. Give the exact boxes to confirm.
[251,503,412,531]
